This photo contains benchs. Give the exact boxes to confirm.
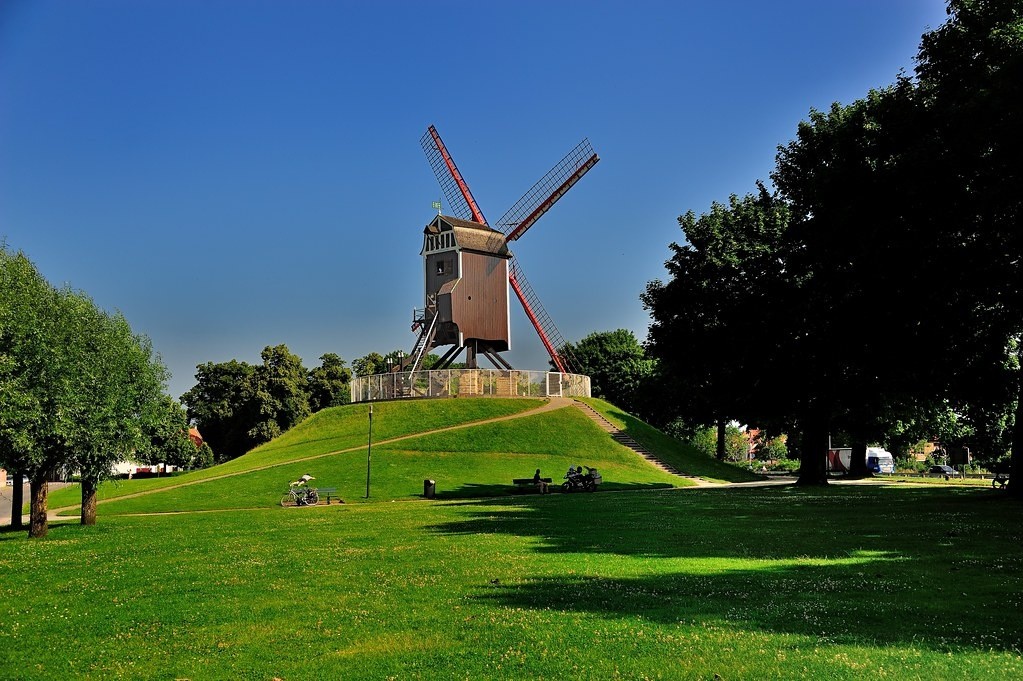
[513,478,552,493]
[291,488,338,505]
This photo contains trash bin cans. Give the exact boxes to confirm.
[424,480,435,498]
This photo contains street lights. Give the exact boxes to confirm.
[962,446,969,464]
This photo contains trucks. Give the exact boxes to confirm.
[827,446,895,474]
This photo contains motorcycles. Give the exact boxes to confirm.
[560,465,603,493]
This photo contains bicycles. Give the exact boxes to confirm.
[280,479,319,508]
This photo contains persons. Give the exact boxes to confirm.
[532,469,549,494]
[288,473,316,487]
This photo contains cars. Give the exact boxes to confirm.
[929,465,962,479]
